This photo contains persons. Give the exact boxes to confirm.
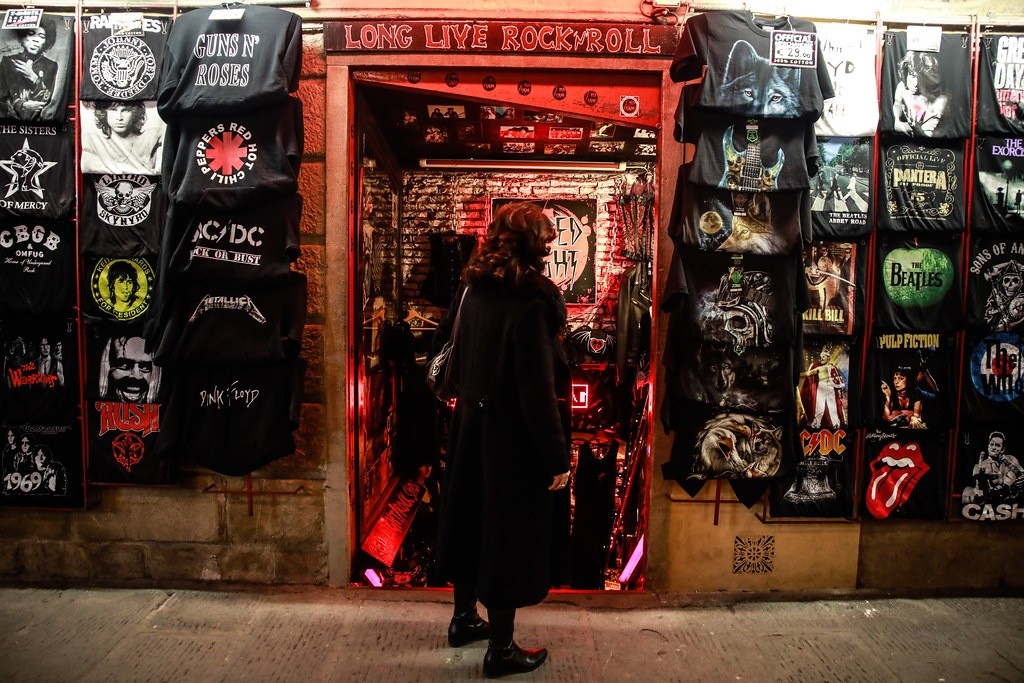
[812,172,859,201]
[480,107,513,119]
[0,18,57,120]
[4,336,64,386]
[802,241,851,308]
[799,352,845,428]
[428,203,572,677]
[85,98,163,174]
[881,349,938,430]
[431,108,458,118]
[893,51,957,137]
[101,261,143,310]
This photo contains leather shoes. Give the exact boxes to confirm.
[483,639,547,677]
[448,606,491,647]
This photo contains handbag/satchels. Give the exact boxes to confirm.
[425,285,470,400]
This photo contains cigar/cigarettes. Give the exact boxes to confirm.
[882,380,888,386]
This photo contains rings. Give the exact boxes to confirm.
[559,484,564,487]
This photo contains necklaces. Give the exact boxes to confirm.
[110,137,134,162]
